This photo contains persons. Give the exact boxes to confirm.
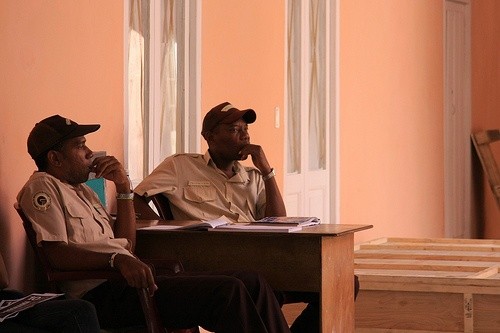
[17,114,291,333]
[0,189,100,333]
[132,99,361,333]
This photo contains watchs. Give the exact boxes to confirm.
[262,168,276,181]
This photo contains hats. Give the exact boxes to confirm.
[27,114,101,160]
[202,101,257,132]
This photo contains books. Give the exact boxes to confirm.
[257,216,321,227]
[138,220,303,233]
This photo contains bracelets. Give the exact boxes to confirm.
[115,192,134,200]
[110,252,120,270]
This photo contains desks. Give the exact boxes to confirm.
[133,221,372,333]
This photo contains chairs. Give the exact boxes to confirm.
[15,203,162,333]
